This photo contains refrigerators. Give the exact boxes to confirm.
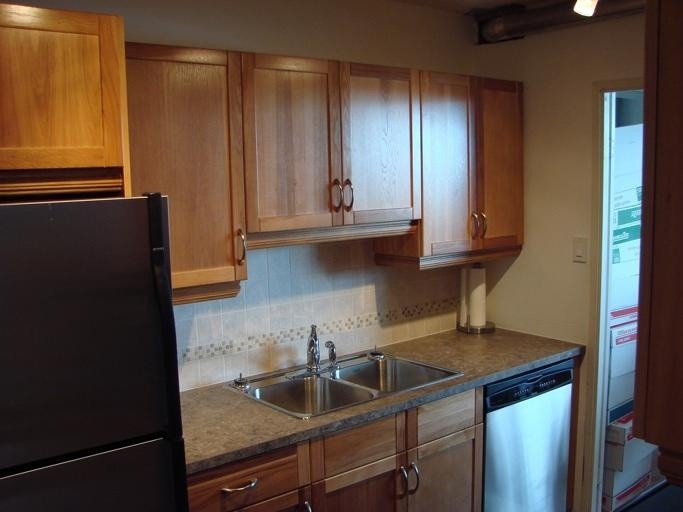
[0,191,190,512]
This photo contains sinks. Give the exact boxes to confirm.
[331,356,464,392]
[245,375,374,421]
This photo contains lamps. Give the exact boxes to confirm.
[573,0,599,17]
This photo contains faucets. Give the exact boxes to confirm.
[325,341,337,366]
[307,324,320,372]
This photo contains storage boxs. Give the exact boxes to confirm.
[603,438,665,511]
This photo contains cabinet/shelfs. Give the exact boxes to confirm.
[370,73,523,274]
[0,5,126,200]
[128,38,246,307]
[241,52,422,247]
[187,389,483,512]
